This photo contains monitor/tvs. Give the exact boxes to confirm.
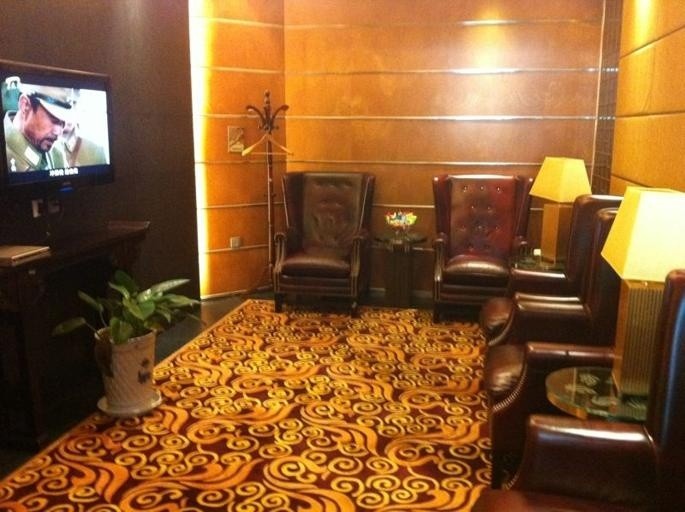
[0,70,116,194]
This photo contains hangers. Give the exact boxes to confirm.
[240,123,293,158]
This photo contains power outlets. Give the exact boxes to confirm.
[230,236,242,248]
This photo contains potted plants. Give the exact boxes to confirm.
[49,270,212,418]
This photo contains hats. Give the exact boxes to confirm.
[19,83,73,121]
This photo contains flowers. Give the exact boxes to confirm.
[385,211,417,236]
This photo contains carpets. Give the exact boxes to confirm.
[1,296,493,511]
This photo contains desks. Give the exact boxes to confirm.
[375,230,426,307]
[0,219,152,449]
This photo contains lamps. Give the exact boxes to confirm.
[602,186,685,396]
[530,157,596,267]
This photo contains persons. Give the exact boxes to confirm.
[4,82,106,173]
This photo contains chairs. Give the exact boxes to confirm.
[430,174,533,322]
[483,211,631,478]
[273,171,373,318]
[479,190,623,335]
[471,269,684,509]
